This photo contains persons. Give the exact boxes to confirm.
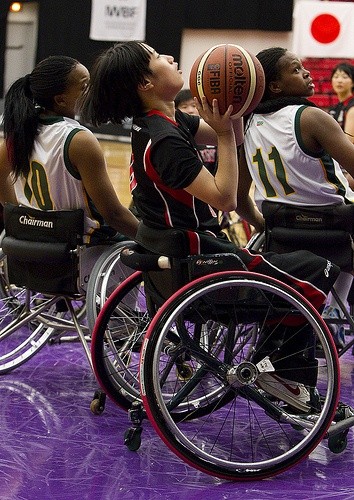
[74,40,341,415]
[174,47,354,349]
[0,56,141,340]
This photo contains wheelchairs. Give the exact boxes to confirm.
[0,201,354,409]
[90,244,354,481]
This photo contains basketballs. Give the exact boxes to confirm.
[190,43,266,119]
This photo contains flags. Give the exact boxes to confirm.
[290,0,353,59]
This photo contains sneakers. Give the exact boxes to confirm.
[255,368,313,414]
[322,307,347,349]
